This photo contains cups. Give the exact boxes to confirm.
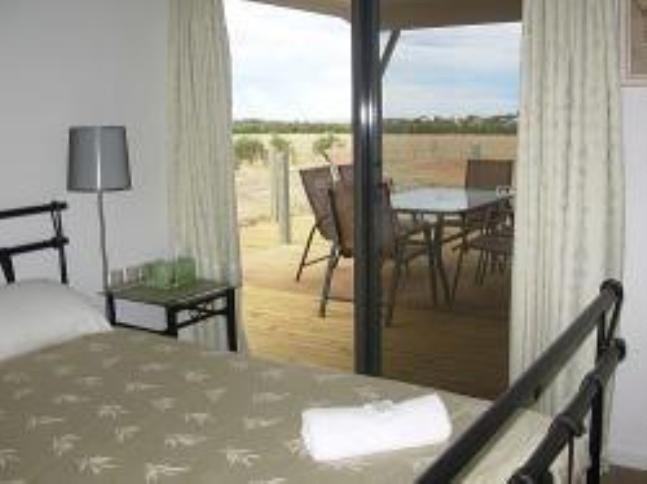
[111,255,195,290]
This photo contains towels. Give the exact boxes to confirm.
[299,392,452,463]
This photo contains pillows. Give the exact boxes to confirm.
[1,277,114,366]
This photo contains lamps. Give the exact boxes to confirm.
[66,123,133,295]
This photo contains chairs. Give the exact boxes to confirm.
[297,158,517,330]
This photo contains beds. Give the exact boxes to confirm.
[0,198,627,484]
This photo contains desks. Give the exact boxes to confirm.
[105,274,236,351]
